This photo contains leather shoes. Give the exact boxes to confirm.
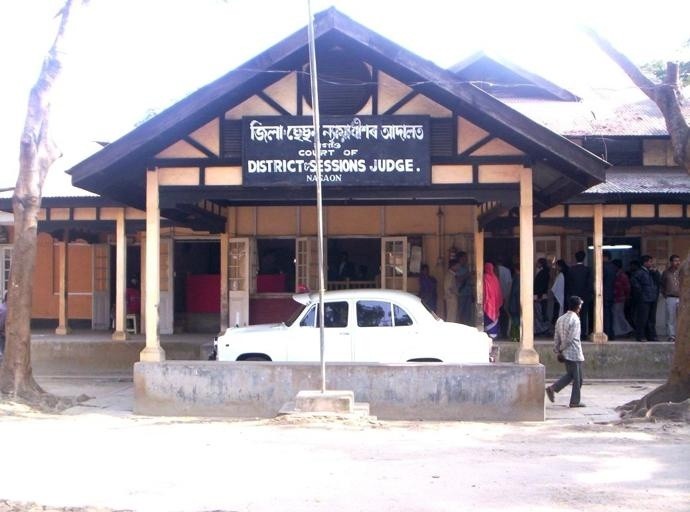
[640,338,659,341]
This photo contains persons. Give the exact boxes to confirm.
[336,252,355,281]
[545,296,586,407]
[1,292,7,358]
[443,250,682,341]
[418,265,437,313]
[112,283,140,332]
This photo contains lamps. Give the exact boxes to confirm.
[588,243,633,251]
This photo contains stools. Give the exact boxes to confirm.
[125,314,140,335]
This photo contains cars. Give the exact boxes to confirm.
[211,289,500,362]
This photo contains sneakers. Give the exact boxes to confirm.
[546,387,554,402]
[569,402,585,407]
